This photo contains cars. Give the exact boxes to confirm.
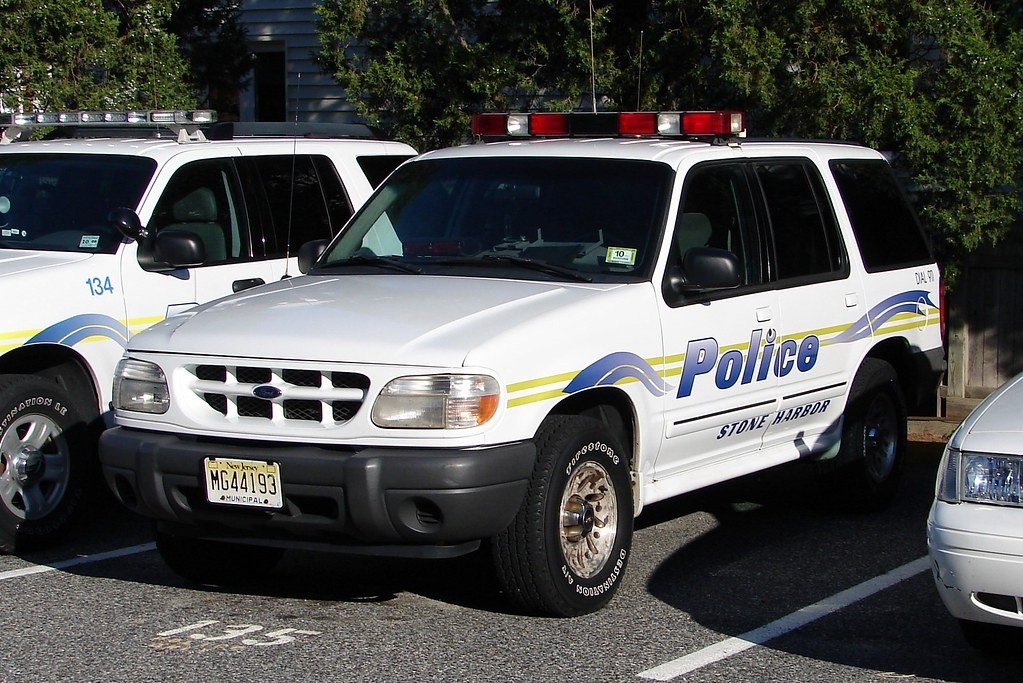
[925,371,1023,629]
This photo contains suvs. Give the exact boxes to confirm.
[1,106,446,560]
[96,108,949,620]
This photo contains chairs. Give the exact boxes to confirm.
[156,186,226,261]
[472,183,547,247]
